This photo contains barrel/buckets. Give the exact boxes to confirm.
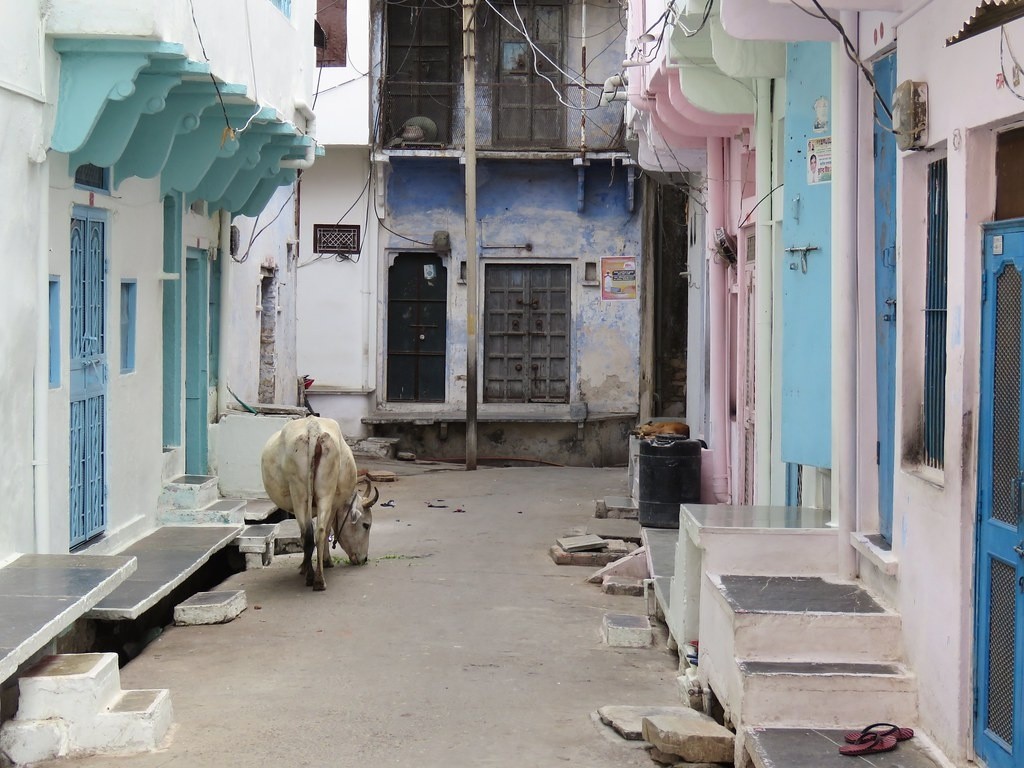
[637,433,702,531]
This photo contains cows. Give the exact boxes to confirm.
[261,415,379,591]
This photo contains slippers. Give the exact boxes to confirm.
[839,733,897,755]
[844,723,914,744]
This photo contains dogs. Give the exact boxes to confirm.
[631,421,689,440]
